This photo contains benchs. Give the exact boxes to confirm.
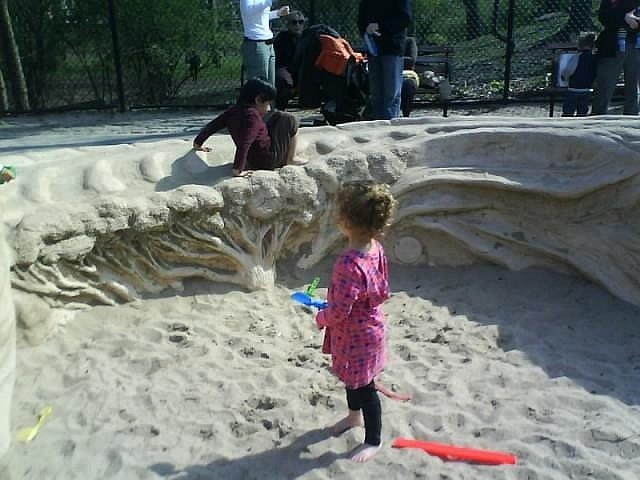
[540,42,625,117]
[234,43,456,117]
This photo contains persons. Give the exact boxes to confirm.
[0,166,17,184]
[315,181,396,464]
[591,0,640,115]
[561,30,595,116]
[239,0,418,127]
[193,75,310,177]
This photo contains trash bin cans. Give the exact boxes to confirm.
[551,46,580,87]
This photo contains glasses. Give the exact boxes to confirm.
[290,20,305,25]
